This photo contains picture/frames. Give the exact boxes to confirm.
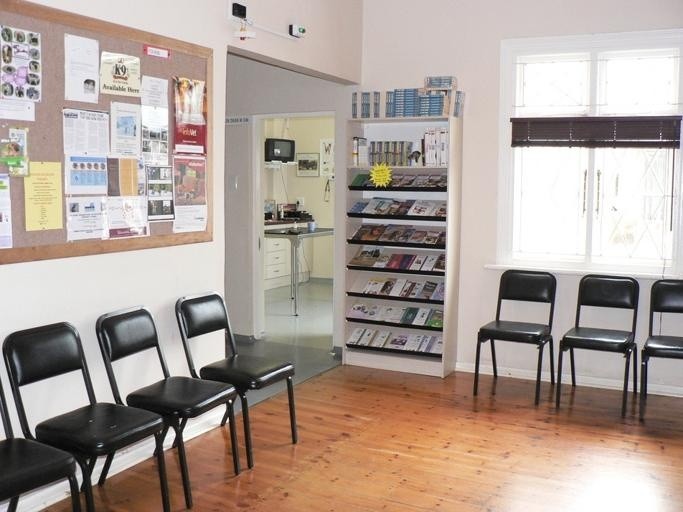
[296,152,320,178]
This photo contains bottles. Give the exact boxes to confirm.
[280,208,284,219]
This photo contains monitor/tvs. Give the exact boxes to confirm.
[264,138,295,163]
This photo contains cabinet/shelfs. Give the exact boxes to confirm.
[338,117,463,378]
[264,238,290,281]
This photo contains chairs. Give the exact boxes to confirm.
[640,277,683,420]
[95,306,242,508]
[173,290,299,469]
[557,272,640,418]
[1,381,81,511]
[1,322,171,511]
[474,268,559,409]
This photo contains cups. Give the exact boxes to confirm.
[308,221,316,232]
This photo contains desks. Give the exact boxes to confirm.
[264,226,334,315]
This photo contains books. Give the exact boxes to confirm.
[352,127,449,167]
[352,76,463,117]
[346,173,447,357]
